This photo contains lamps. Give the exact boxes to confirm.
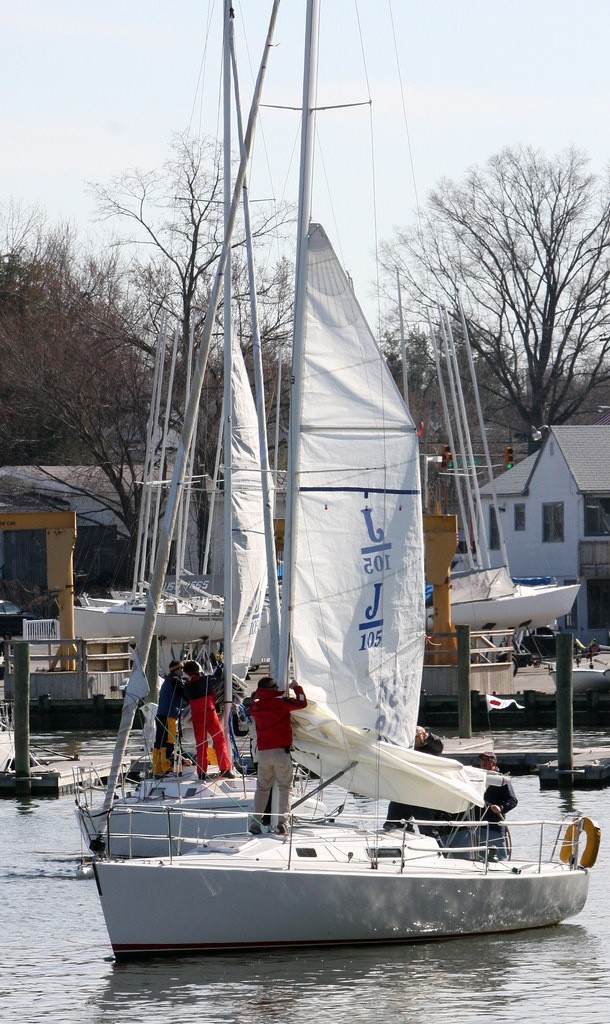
[531,424,551,440]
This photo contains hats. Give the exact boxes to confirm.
[169,659,184,672]
[258,677,279,689]
[183,660,202,676]
[478,751,496,762]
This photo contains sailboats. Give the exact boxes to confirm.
[65,1,599,954]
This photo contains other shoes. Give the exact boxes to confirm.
[164,770,182,778]
[248,825,261,834]
[219,770,235,779]
[277,822,289,835]
[198,774,206,782]
[154,775,167,780]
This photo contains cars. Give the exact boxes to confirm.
[0,597,41,640]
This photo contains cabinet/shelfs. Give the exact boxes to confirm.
[83,635,136,701]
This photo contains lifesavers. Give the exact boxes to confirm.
[559,817,600,867]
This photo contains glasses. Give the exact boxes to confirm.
[480,757,494,763]
[415,731,423,737]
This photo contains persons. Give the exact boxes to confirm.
[243,677,307,835]
[383,726,445,831]
[149,660,234,778]
[467,750,518,862]
